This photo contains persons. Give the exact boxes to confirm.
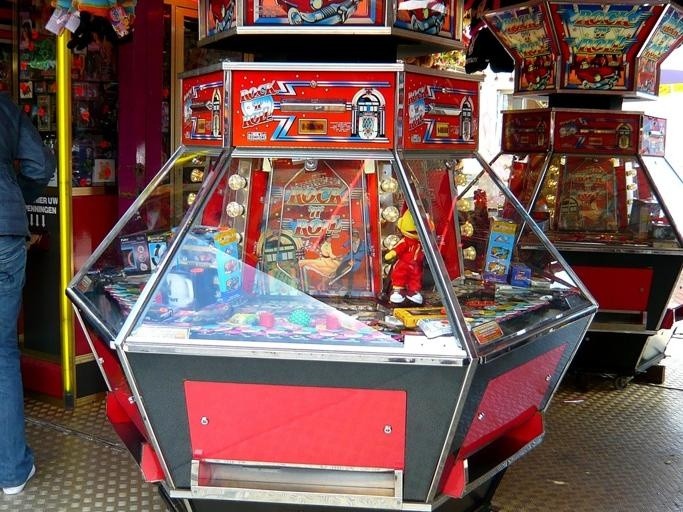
[335,230,371,297]
[103,162,112,179]
[38,97,48,125]
[0,90,56,494]
[383,200,426,304]
[21,222,52,352]
[298,233,342,292]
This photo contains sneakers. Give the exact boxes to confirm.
[3,463,36,496]
